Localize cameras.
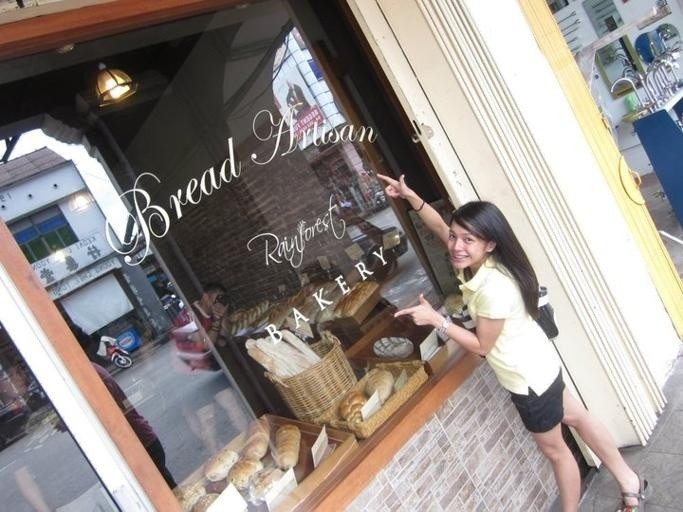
[214,294,231,306]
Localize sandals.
[615,471,653,512]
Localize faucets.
[609,32,678,109]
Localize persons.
[283,77,310,122]
[48,320,185,502]
[373,170,654,511]
[169,280,248,453]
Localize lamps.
[95,59,139,111]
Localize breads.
[366,370,395,407]
[372,335,415,359]
[173,418,301,512]
[253,280,383,336]
[338,391,367,424]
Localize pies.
[227,299,270,337]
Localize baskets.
[263,329,360,425]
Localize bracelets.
[414,200,426,213]
[210,324,219,332]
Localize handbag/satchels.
[459,286,558,359]
